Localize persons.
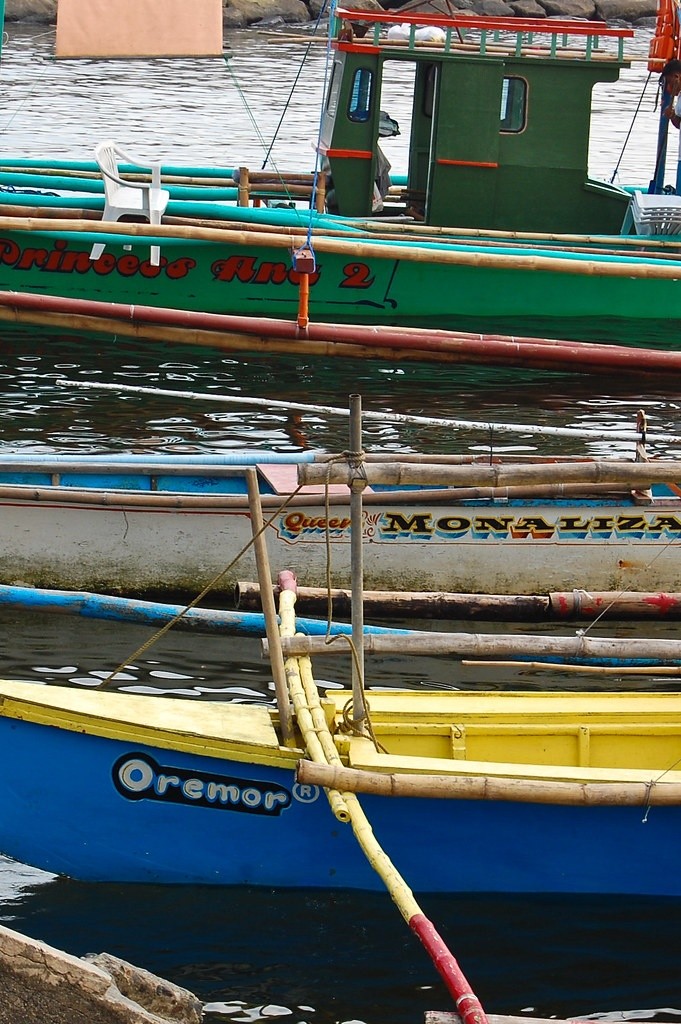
[658,60,681,196]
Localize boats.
[0,451,681,622]
[0,1,681,365]
[0,676,681,901]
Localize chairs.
[85,142,170,267]
[620,189,680,235]
